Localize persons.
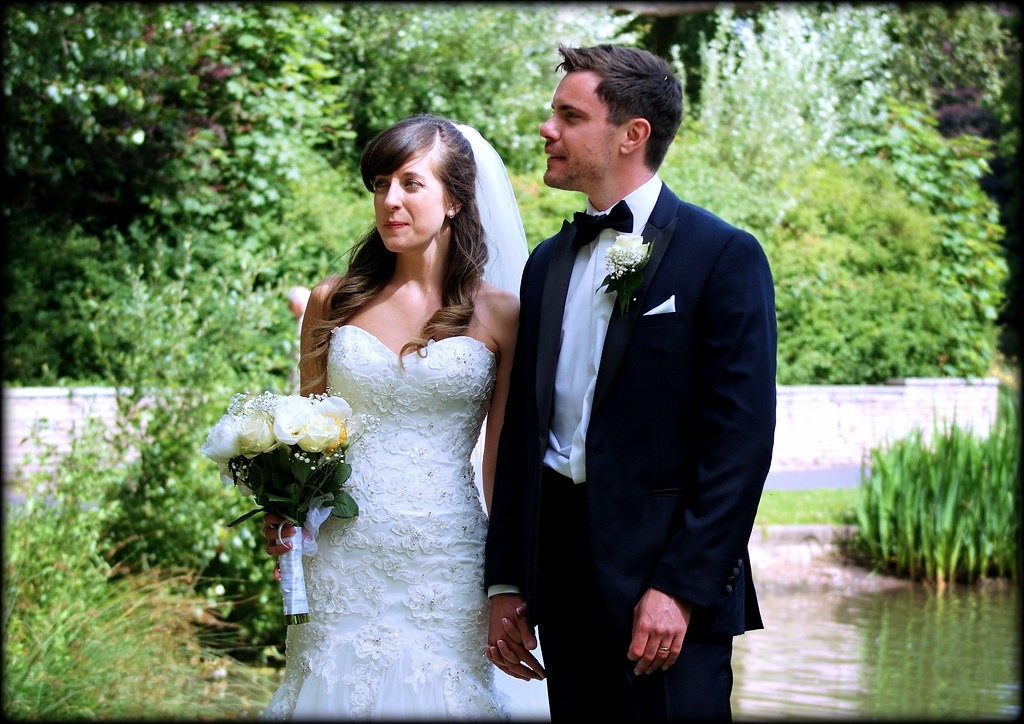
[485,45,777,723]
[266,113,534,724]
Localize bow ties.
[573,200,633,246]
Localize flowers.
[202,389,366,626]
[594,234,653,317]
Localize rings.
[659,647,669,653]
